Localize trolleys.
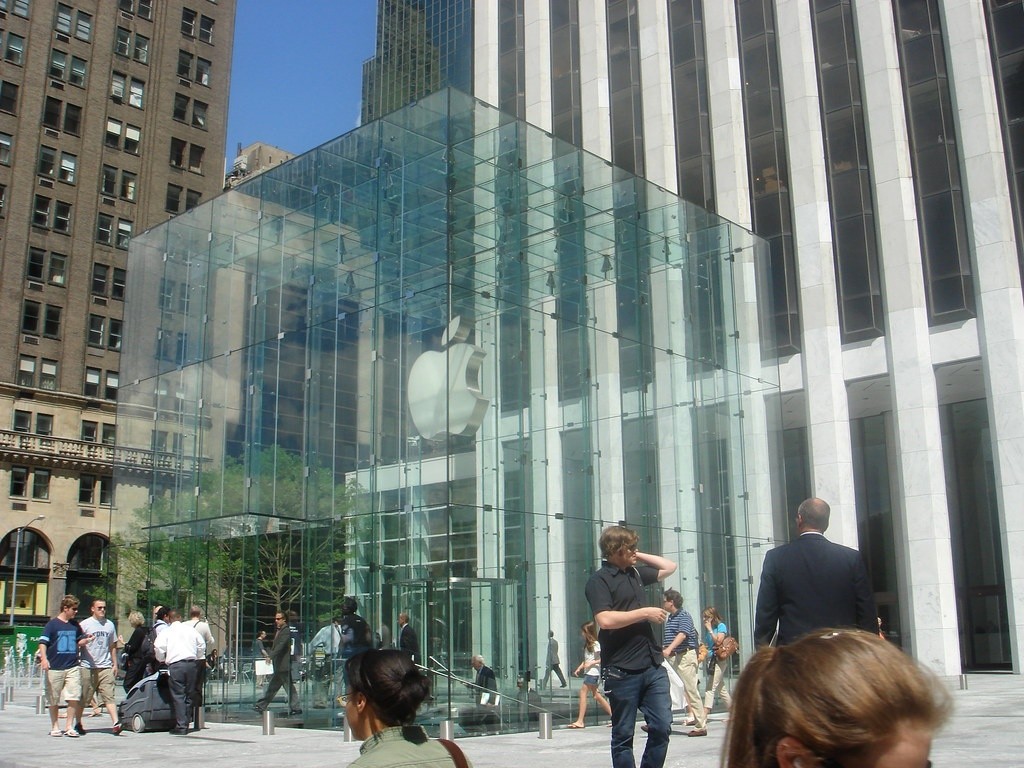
[115,663,176,733]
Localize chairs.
[236,663,253,685]
[221,663,238,684]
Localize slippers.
[566,723,585,728]
[606,723,612,727]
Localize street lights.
[8,514,47,626]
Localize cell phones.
[707,617,714,622]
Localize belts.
[669,647,693,656]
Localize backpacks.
[140,623,166,656]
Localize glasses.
[336,690,371,707]
[95,606,107,611]
[68,607,80,612]
[275,618,282,621]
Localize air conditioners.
[110,88,122,98]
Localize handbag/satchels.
[121,643,132,670]
[712,622,738,661]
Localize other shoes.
[481,730,500,736]
[684,716,694,723]
[64,728,79,737]
[169,728,188,735]
[688,729,707,737]
[468,732,481,736]
[249,705,265,715]
[687,720,707,725]
[286,710,302,716]
[560,683,567,687]
[313,705,326,708]
[88,707,102,717]
[74,723,86,734]
[113,721,128,736]
[641,725,648,732]
[49,729,62,736]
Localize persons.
[118,605,215,735]
[250,611,303,715]
[250,630,268,685]
[753,498,879,653]
[462,655,497,731]
[719,628,955,768]
[542,630,567,688]
[339,596,370,685]
[567,621,612,729]
[58,693,101,717]
[206,650,226,671]
[74,598,123,736]
[400,612,421,664]
[640,590,707,737]
[511,676,542,732]
[702,607,732,723]
[39,595,95,736]
[584,526,672,768]
[310,617,343,706]
[340,649,473,768]
